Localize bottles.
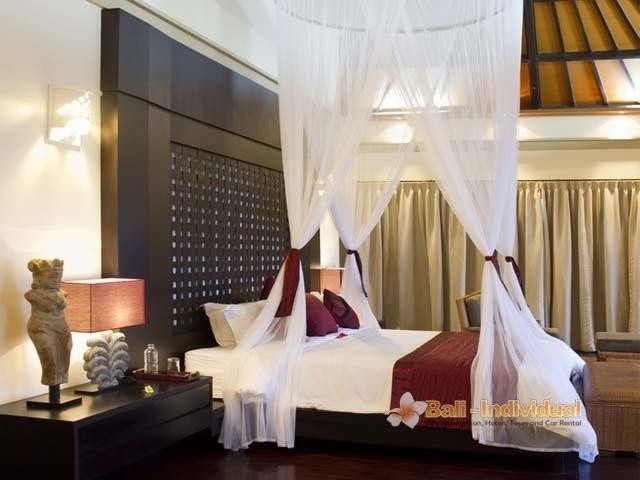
[144,343,158,372]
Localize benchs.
[586,357,640,454]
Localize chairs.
[455,291,561,340]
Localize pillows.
[223,296,270,344]
[321,287,361,330]
[201,299,238,348]
[303,292,340,337]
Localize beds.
[101,8,597,480]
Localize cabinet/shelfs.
[1,368,226,478]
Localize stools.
[595,331,640,361]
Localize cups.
[167,357,181,376]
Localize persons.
[23,258,73,387]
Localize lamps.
[59,275,147,398]
[45,79,97,151]
[318,267,344,292]
